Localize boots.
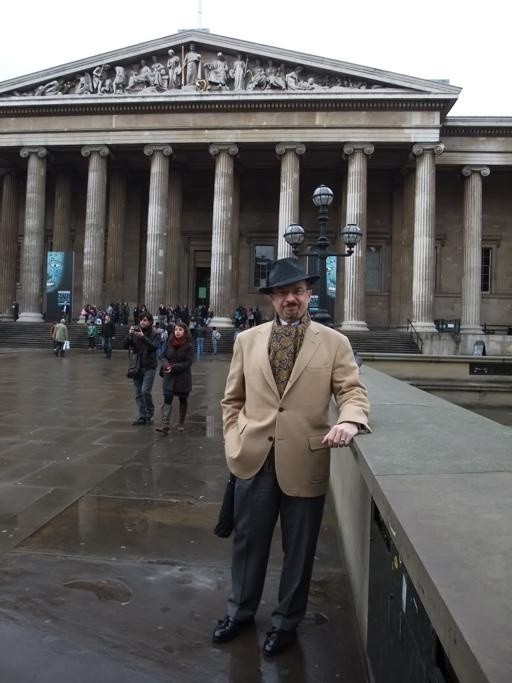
[155,405,172,434]
[176,404,186,432]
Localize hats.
[258,257,321,294]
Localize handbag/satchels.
[126,352,142,379]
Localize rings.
[340,438,345,441]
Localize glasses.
[276,288,306,296]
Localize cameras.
[134,326,141,332]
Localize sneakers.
[132,415,153,426]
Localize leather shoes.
[212,616,245,644]
[262,627,298,657]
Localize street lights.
[283,183,365,328]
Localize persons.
[51,302,261,360]
[39,43,329,97]
[123,311,161,427]
[12,301,19,322]
[212,258,373,657]
[155,323,194,435]
